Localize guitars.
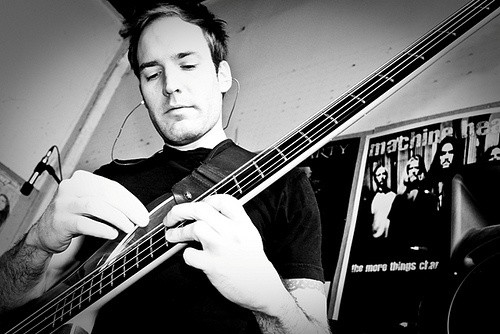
[1,0,499,331]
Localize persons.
[363,136,500,262]
[1,0,332,334]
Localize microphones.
[21,146,54,196]
